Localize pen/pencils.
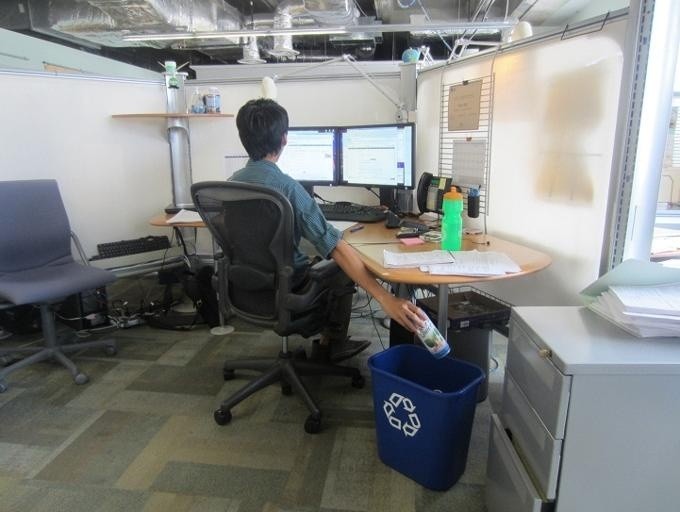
[467,184,482,196]
[350,225,364,233]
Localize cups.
[468,196,480,218]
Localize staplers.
[396,220,429,238]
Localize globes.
[402,46,419,63]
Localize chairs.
[1,177,124,393]
[190,180,367,435]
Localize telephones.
[416,172,453,213]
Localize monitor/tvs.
[274,125,339,198]
[338,121,416,217]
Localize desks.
[149,199,552,342]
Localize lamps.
[447,19,533,60]
[259,52,410,129]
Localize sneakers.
[312,337,371,365]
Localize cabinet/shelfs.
[485,306,680,512]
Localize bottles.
[441,185,464,250]
[191,87,205,113]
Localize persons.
[227,97,429,363]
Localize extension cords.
[117,311,160,329]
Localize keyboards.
[318,201,385,222]
[97,235,171,259]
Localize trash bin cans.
[367,344,486,492]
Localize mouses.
[385,216,400,228]
